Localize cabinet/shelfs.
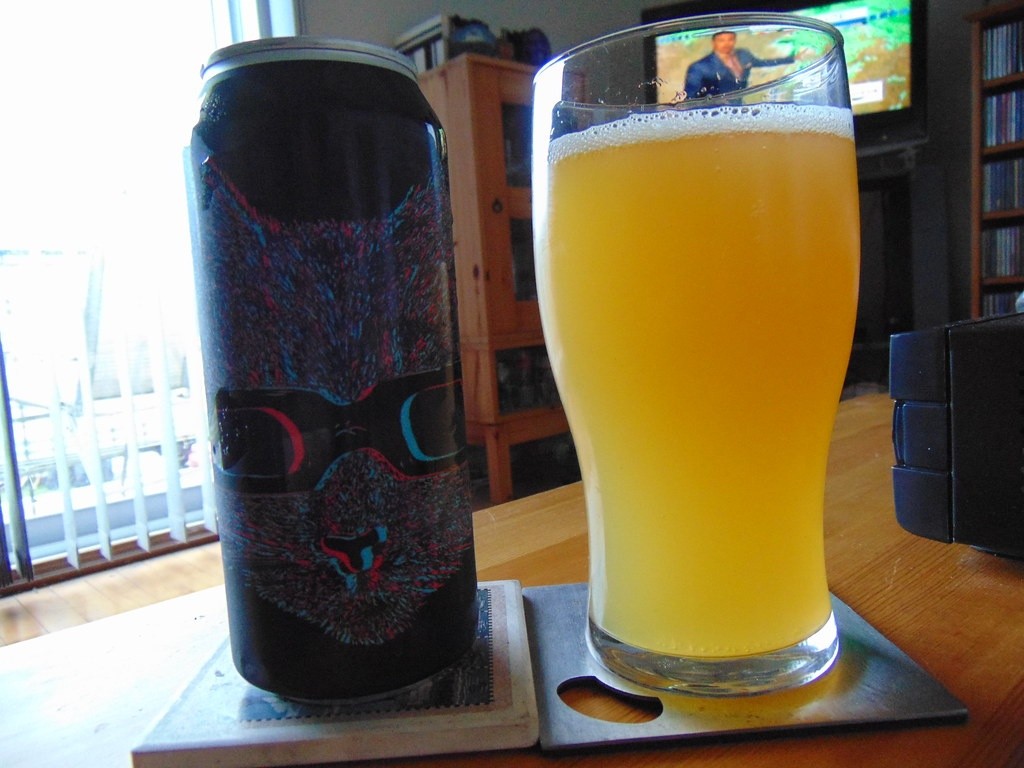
[961,0,1024,318]
[418,52,568,507]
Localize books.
[978,21,1024,317]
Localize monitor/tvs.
[641,0,927,156]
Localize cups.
[527,7,865,699]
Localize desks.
[0,394,1024,768]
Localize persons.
[684,30,809,112]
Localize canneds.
[189,35,478,705]
